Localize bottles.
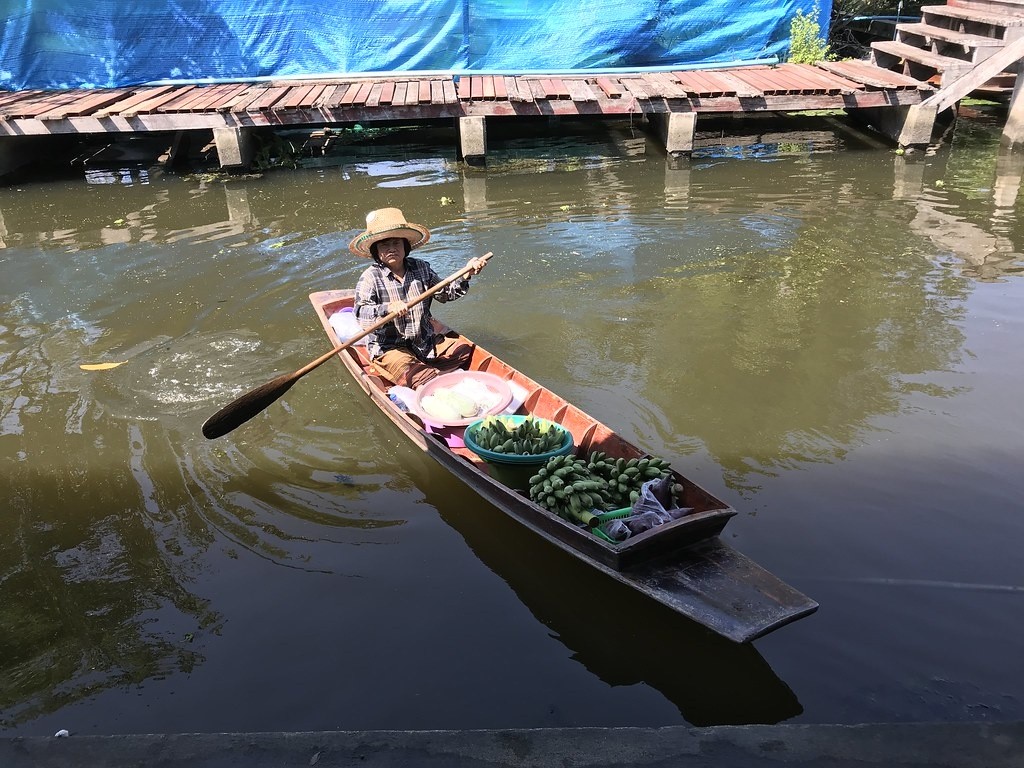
[390,394,410,413]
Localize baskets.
[592,507,632,544]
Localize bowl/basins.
[463,413,574,490]
[416,370,513,429]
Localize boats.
[310,289,821,648]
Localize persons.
[349,208,487,391]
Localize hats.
[349,208,430,259]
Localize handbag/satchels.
[605,478,680,542]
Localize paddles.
[201,250,495,440]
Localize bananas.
[475,411,566,455]
[528,451,676,515]
